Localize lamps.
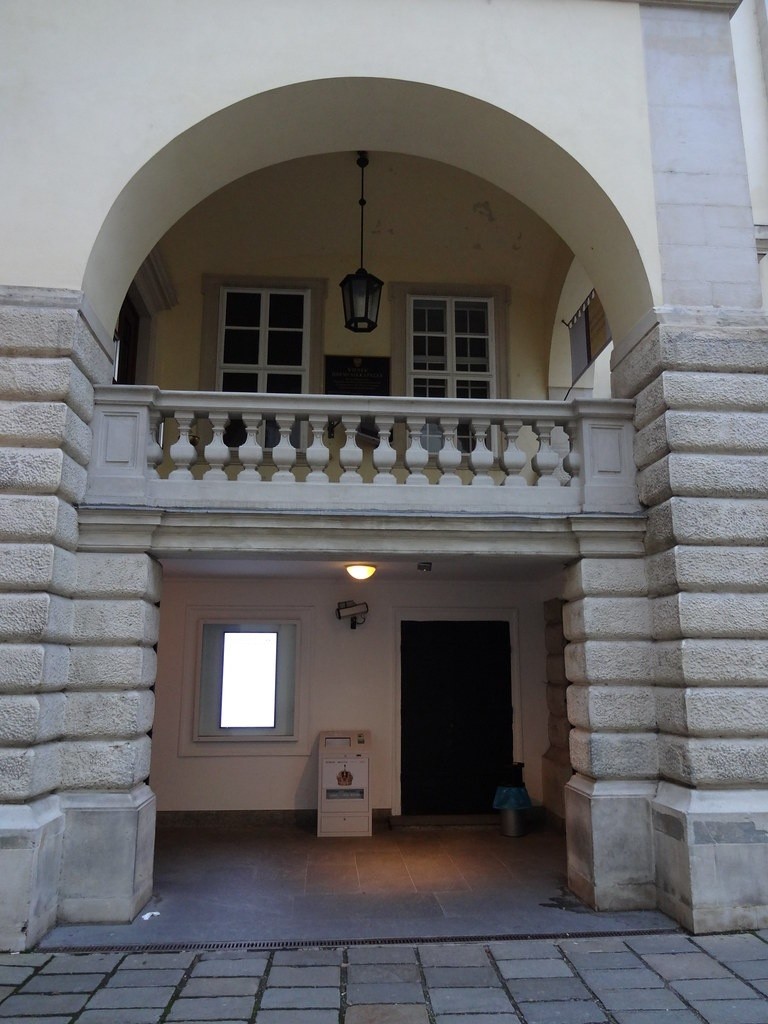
[344,564,377,580]
[340,151,385,333]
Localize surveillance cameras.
[334,601,369,620]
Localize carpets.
[388,815,504,828]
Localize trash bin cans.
[493,785,534,838]
